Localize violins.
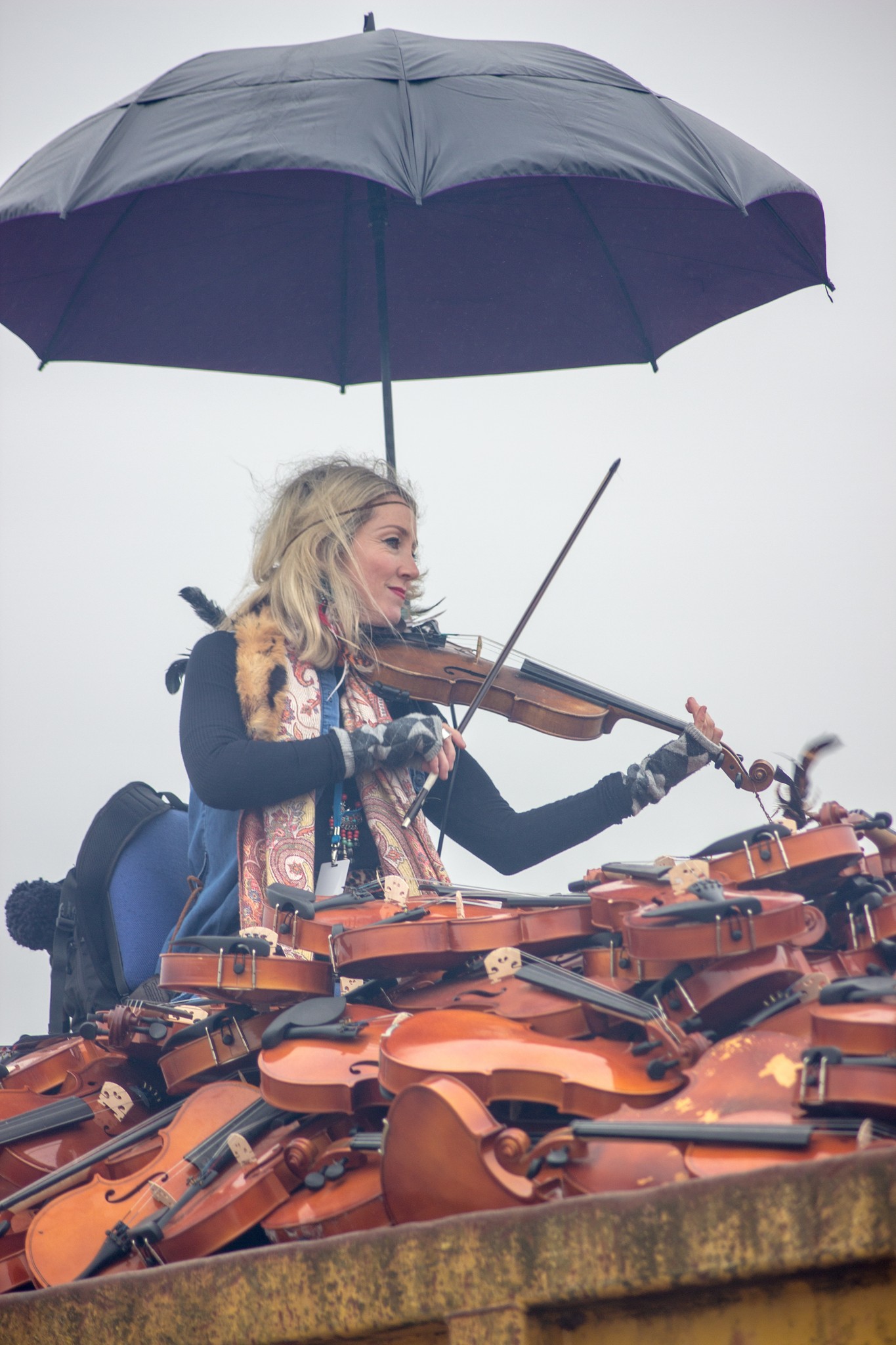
[1,730,894,1294]
[363,632,775,795]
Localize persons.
[156,454,726,971]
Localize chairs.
[47,780,189,1044]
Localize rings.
[442,728,452,740]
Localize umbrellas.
[1,13,835,484]
